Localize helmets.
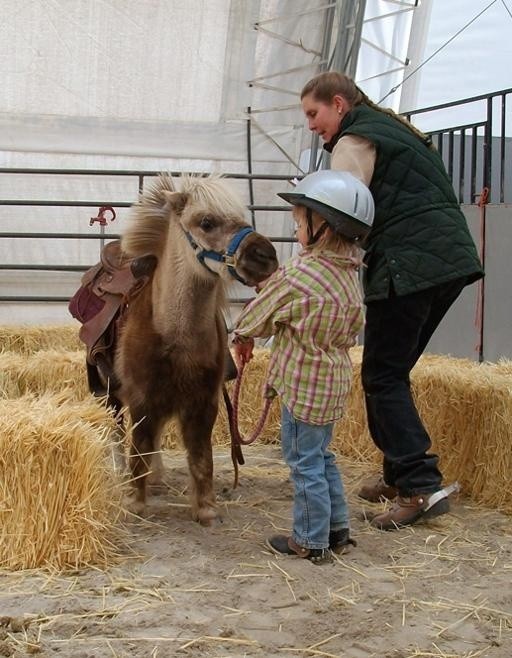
[274,170,376,244]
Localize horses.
[86,168,278,528]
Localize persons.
[229,168,376,563]
[254,70,485,528]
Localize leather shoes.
[356,470,450,531]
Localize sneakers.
[265,522,350,565]
